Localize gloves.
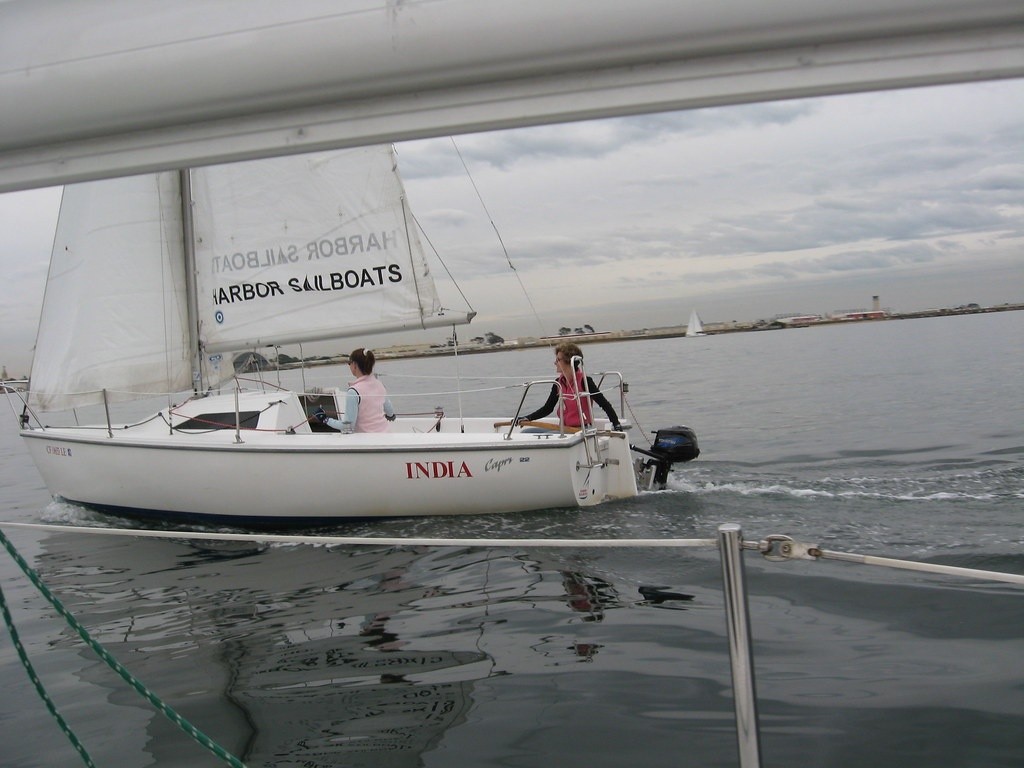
[511,416,529,428]
[615,424,623,432]
[312,407,329,424]
[385,414,396,421]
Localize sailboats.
[0,143,700,517]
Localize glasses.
[556,357,563,362]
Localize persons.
[513,343,623,432]
[313,349,395,433]
